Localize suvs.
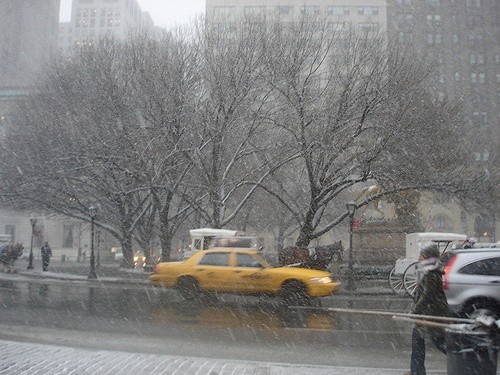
[431,247,500,343]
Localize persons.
[306,253,323,271]
[464,240,472,249]
[404,243,453,375]
[41,240,52,271]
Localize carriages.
[188,227,345,276]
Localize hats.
[420,245,440,259]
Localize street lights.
[88,204,99,278]
[26,215,38,270]
[346,198,357,291]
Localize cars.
[146,247,343,309]
